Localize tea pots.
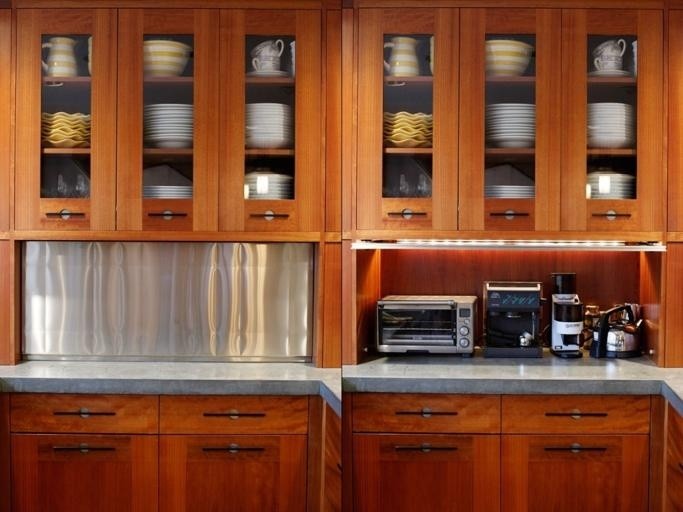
[41,38,80,76]
[589,305,645,358]
[383,37,420,78]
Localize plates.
[588,70,630,76]
[486,181,534,195]
[586,171,632,197]
[246,70,289,78]
[244,171,296,199]
[244,104,294,149]
[146,181,190,200]
[145,103,194,151]
[587,102,632,147]
[384,110,435,147]
[485,104,535,148]
[44,106,93,147]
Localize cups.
[250,39,285,70]
[592,39,627,70]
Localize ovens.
[377,294,477,357]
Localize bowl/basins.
[485,39,534,78]
[142,40,192,77]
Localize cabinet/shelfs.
[341,347,683,512]
[2,2,342,241]
[0,361,341,512]
[342,1,682,244]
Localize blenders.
[551,270,584,359]
